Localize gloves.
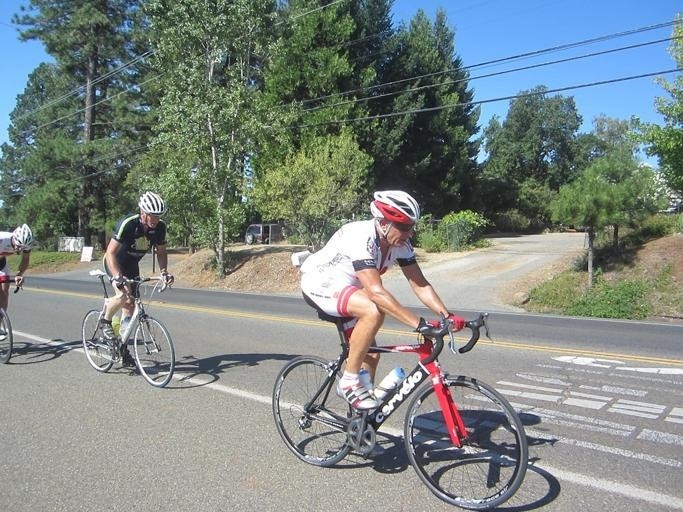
[427,319,441,328]
[448,313,465,329]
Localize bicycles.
[82,269,175,388]
[0,280,24,363]
[272,309,528,511]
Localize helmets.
[12,223,33,251]
[138,191,166,216]
[370,190,421,225]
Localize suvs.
[244,223,284,244]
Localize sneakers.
[123,355,136,366]
[99,319,115,339]
[361,440,385,455]
[337,380,379,409]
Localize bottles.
[119,315,132,338]
[358,367,373,397]
[373,366,408,400]
[111,313,120,336]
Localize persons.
[300,189,466,457]
[99,191,175,371]
[0,223,35,342]
